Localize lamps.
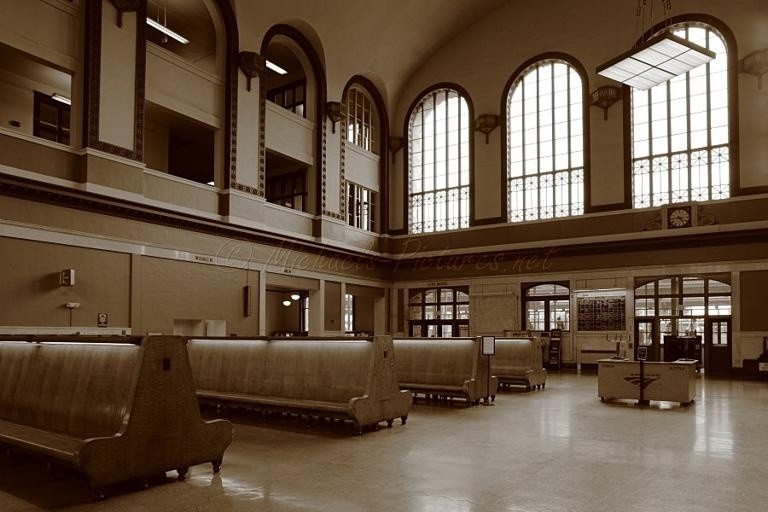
[326,101,348,135]
[239,51,263,92]
[474,113,499,144]
[388,136,405,164]
[593,2,716,123]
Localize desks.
[575,342,625,376]
[594,357,699,407]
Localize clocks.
[665,204,692,230]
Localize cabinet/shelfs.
[664,334,703,372]
